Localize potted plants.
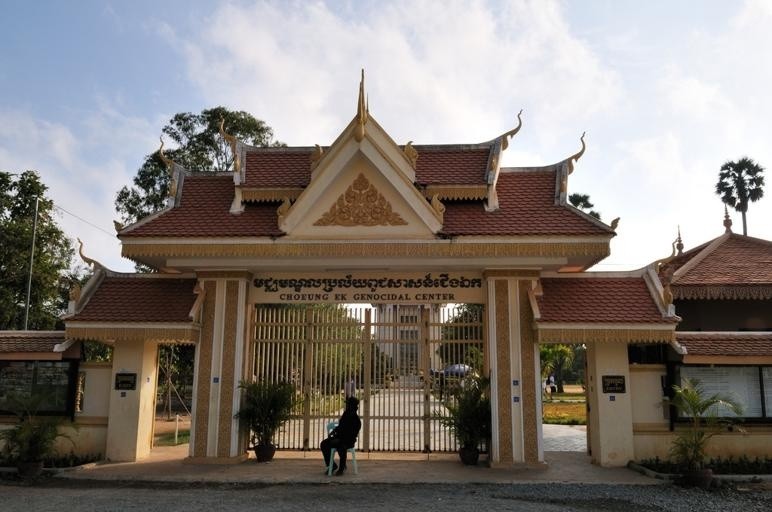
[428,375,490,465]
[0,388,80,477]
[232,378,306,461]
[654,378,748,489]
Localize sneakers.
[337,465,346,476]
[326,464,338,475]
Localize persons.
[550,372,556,400]
[542,376,548,400]
[345,376,354,398]
[436,373,449,403]
[320,397,361,477]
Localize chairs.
[323,422,357,476]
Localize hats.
[343,397,360,410]
